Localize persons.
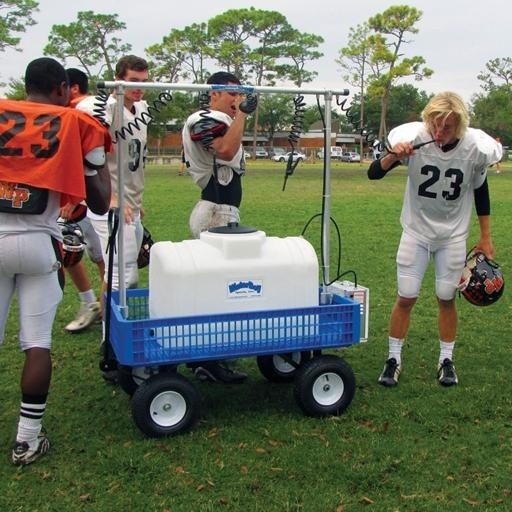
[367,89,504,387]
[59,67,104,332]
[87,56,152,342]
[495,137,500,174]
[0,55,112,467]
[181,71,249,385]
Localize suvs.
[316,146,344,160]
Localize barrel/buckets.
[148,221,320,350]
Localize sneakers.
[376,357,403,387]
[193,361,249,386]
[10,432,53,466]
[435,356,459,386]
[64,296,103,332]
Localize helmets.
[55,219,89,268]
[457,251,505,308]
[135,227,157,270]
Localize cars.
[339,151,360,164]
[242,145,307,162]
[503,146,512,160]
